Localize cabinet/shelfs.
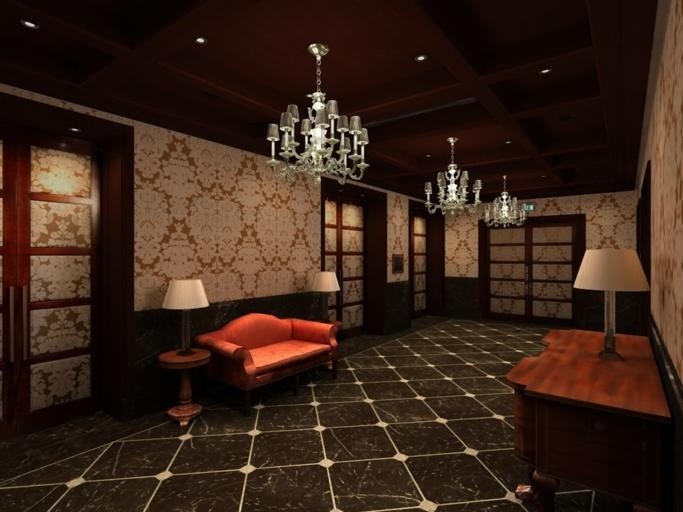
[505,328,671,501]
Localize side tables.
[158,349,210,421]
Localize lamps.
[161,278,210,356]
[267,41,371,186]
[308,271,341,324]
[423,137,482,218]
[573,249,651,363]
[479,174,526,229]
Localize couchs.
[193,312,339,419]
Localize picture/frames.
[392,254,404,273]
